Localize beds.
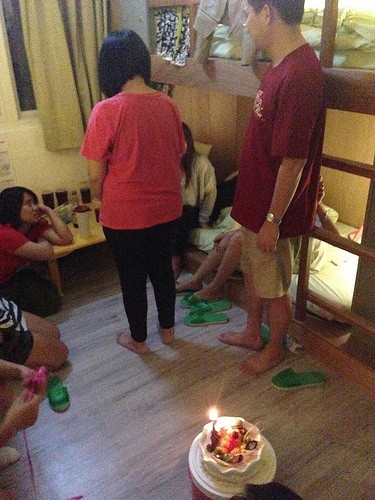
[147,1,375,399]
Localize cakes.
[196,417,266,483]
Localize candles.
[207,408,217,431]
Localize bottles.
[75,206,92,238]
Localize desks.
[47,202,107,297]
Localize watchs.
[266,213,281,224]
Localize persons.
[216,0,328,375]
[0,297,69,372]
[175,175,340,302]
[0,360,47,469]
[170,121,217,280]
[0,186,73,318]
[79,29,188,355]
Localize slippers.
[271,367,327,390]
[175,280,232,326]
[46,375,71,412]
[261,325,270,343]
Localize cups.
[80,187,92,204]
[55,188,68,206]
[70,205,79,229]
[41,190,55,210]
